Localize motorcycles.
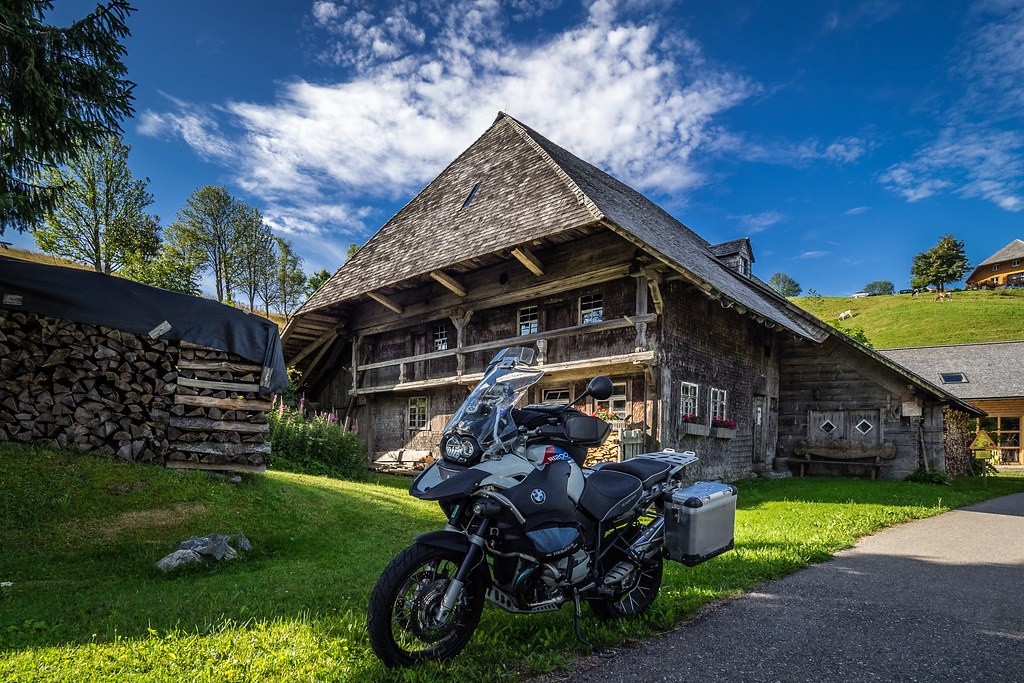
[366,346,737,672]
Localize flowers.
[593,406,619,420]
[682,413,707,426]
[713,416,736,429]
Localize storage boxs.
[661,482,738,568]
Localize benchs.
[788,440,896,481]
